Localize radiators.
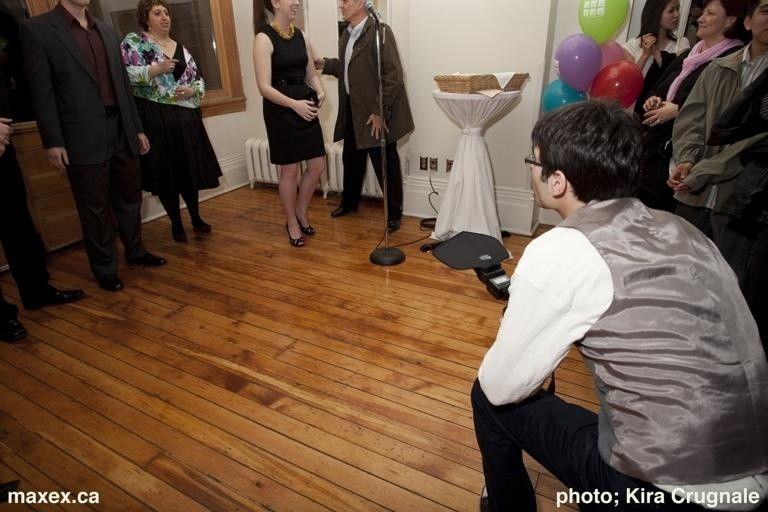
[245,137,407,199]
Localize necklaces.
[150,32,167,47]
[270,21,295,41]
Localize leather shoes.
[388,220,400,233]
[286,222,304,247]
[127,253,167,266]
[331,205,359,217]
[295,217,314,236]
[96,274,124,291]
[21,287,84,310]
[173,219,211,241]
[0,298,29,341]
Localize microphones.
[364,0,384,27]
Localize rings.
[181,91,184,95]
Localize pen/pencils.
[163,53,170,59]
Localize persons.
[621,0,690,117]
[638,1,746,204]
[118,0,223,243]
[314,1,414,233]
[25,0,168,291]
[666,1,768,348]
[0,41,84,343]
[470,101,767,509]
[252,0,326,248]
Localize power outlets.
[419,156,428,170]
[430,158,438,171]
[446,159,453,171]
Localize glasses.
[525,153,541,166]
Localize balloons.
[576,0,628,44]
[554,34,599,95]
[540,82,591,114]
[586,38,623,94]
[591,61,646,109]
